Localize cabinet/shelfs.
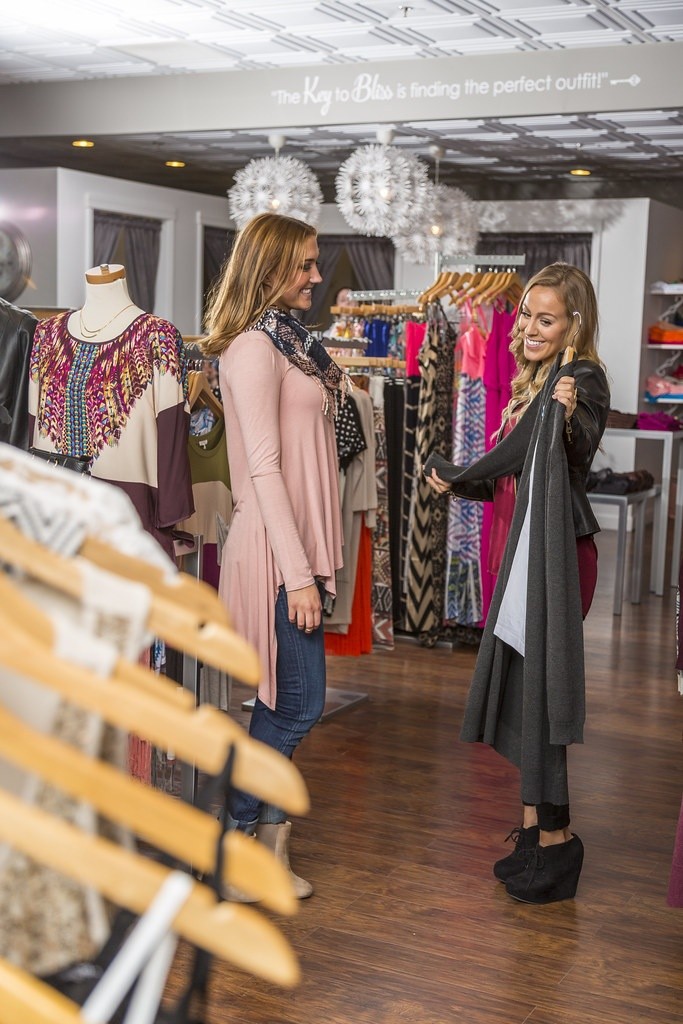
[642,289,683,405]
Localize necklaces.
[80,304,136,338]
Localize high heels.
[493,820,542,884]
[506,835,584,906]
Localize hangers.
[329,255,526,379]
[182,340,224,418]
[558,312,581,367]
[1,456,311,1024]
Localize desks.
[602,429,683,595]
[586,485,661,616]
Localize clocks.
[0,220,32,302]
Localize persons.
[193,214,342,902]
[423,263,611,902]
[336,288,360,318]
[28,264,195,561]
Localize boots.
[207,811,258,903]
[253,821,312,899]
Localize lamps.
[335,128,433,237]
[389,146,479,263]
[227,133,326,233]
